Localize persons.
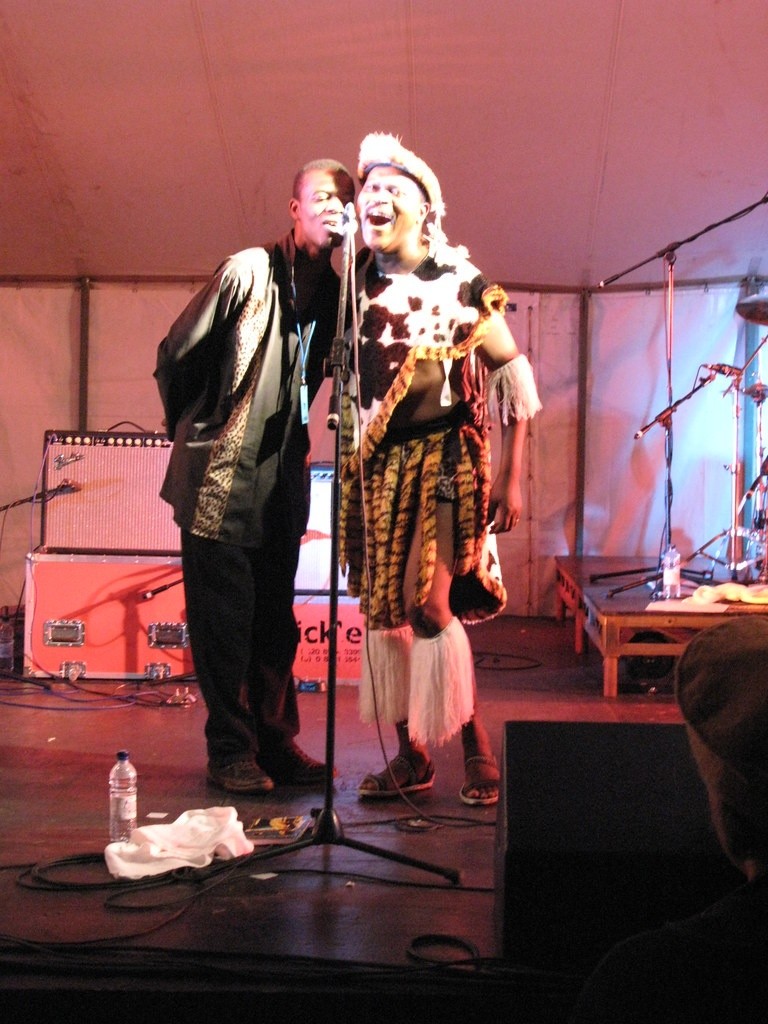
[153,158,355,790]
[335,132,543,806]
[572,618,768,1024]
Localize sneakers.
[256,742,336,788]
[207,757,273,793]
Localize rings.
[516,518,519,522]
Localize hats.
[358,128,445,226]
[675,614,768,794]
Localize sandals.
[359,755,435,796]
[459,752,501,804]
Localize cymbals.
[736,294,768,326]
[721,384,768,395]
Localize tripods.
[587,191,768,599]
[191,223,463,885]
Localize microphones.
[63,478,83,492]
[703,363,741,377]
[336,202,359,236]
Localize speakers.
[40,430,183,557]
[292,464,351,596]
[493,720,749,961]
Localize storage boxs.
[23,429,196,681]
[291,594,366,687]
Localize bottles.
[0,616,14,672]
[663,544,681,598]
[108,750,138,844]
